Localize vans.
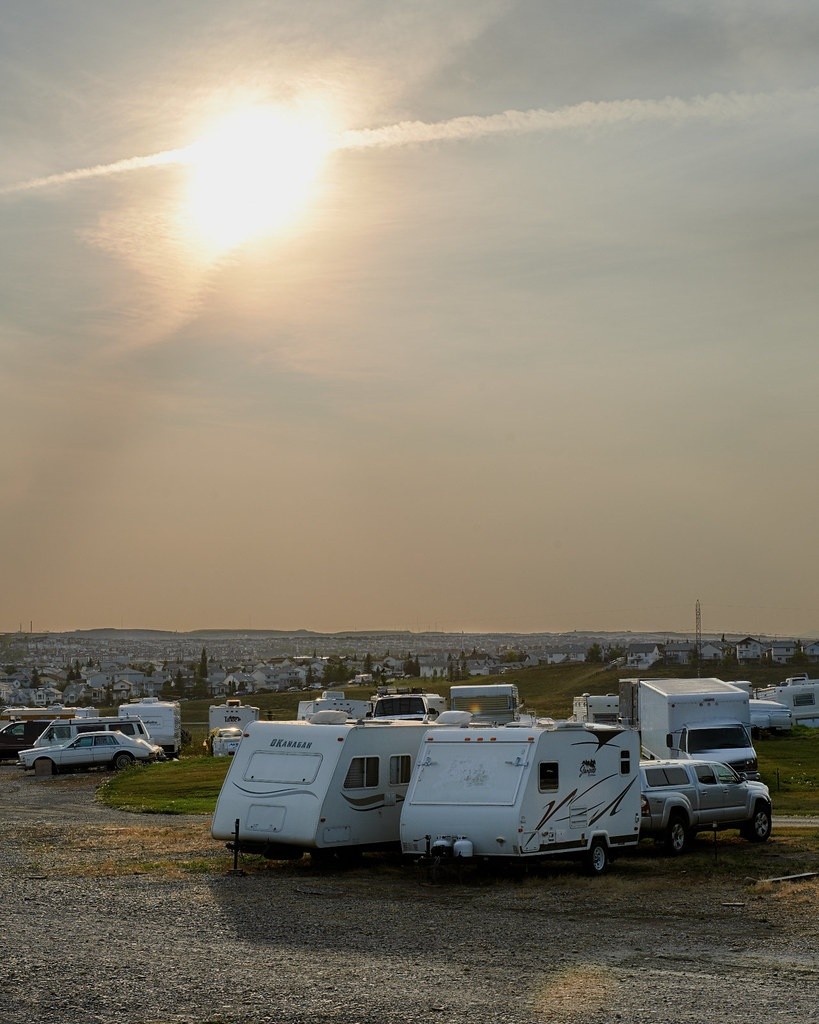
[32,715,150,748]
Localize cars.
[232,691,246,696]
[303,686,314,691]
[348,680,355,684]
[0,720,53,762]
[318,686,329,690]
[16,731,157,775]
[254,688,269,694]
[212,729,243,752]
[214,693,225,698]
[174,698,189,702]
[0,702,64,709]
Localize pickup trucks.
[287,686,303,692]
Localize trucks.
[355,673,374,684]
[366,694,436,721]
[638,678,761,782]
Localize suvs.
[639,759,773,858]
